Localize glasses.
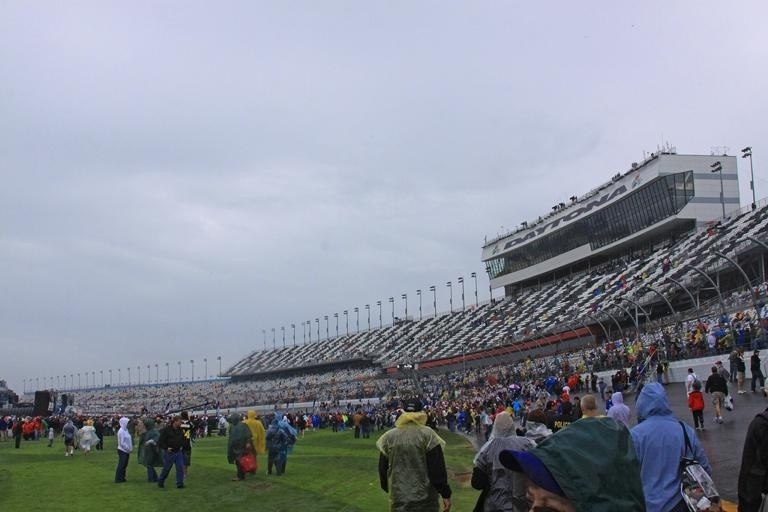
[511,495,560,512]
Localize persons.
[224,305,471,440]
[241,410,265,476]
[265,418,288,476]
[1,413,229,457]
[470,200,766,511]
[115,416,132,483]
[226,409,259,481]
[274,409,299,473]
[157,415,193,487]
[136,417,162,481]
[18,381,224,420]
[375,397,453,512]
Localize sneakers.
[718,418,724,424]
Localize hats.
[498,448,567,495]
[403,398,425,412]
[715,361,721,364]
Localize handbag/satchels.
[674,418,724,512]
[237,448,257,473]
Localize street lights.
[711,160,725,219]
[741,145,756,206]
[260,292,410,353]
[457,276,466,310]
[416,289,422,320]
[23,355,223,394]
[429,285,437,316]
[446,281,454,312]
[471,271,478,307]
[484,266,493,301]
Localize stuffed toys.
[178,410,191,478]
